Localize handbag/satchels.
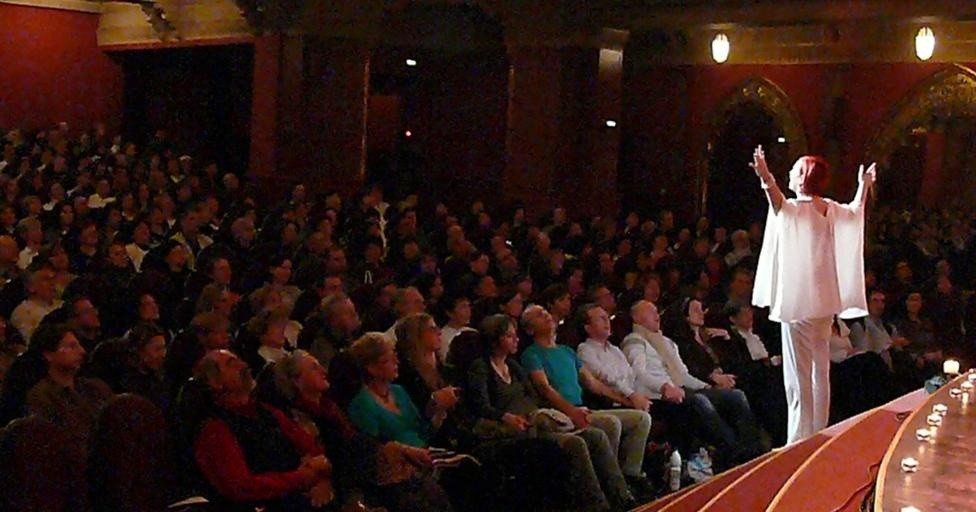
[530,408,576,434]
[646,440,675,476]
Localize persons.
[0,112,975,512]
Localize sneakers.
[618,468,661,509]
[721,439,771,462]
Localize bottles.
[699,446,709,463]
[663,442,683,490]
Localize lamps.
[711,29,735,66]
[912,20,937,64]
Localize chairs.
[0,415,82,512]
[85,392,213,512]
